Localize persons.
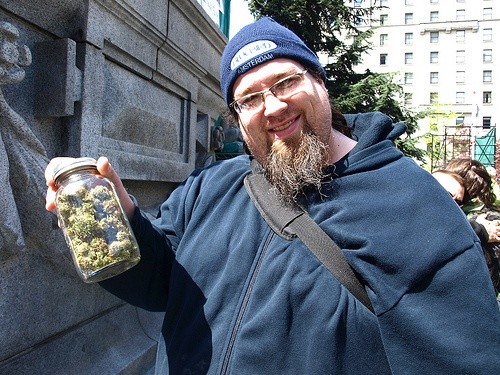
[45,15,498,374]
[429,155,499,244]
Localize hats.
[220,15,327,115]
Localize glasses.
[229,66,311,114]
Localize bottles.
[50,156,141,284]
